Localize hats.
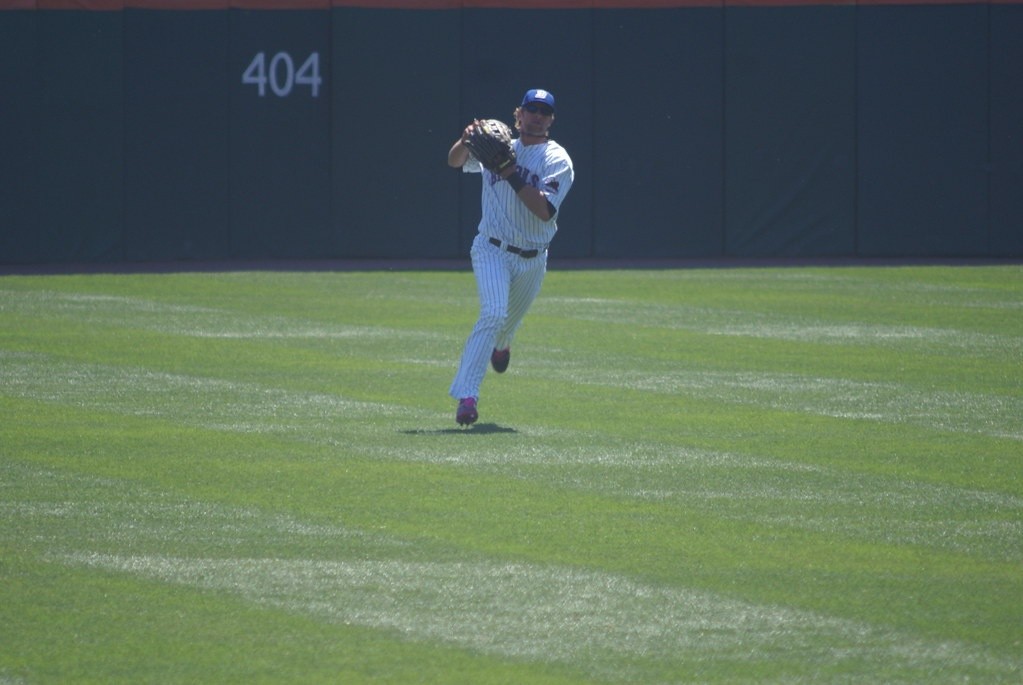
[520,89,556,113]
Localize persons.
[449,89,577,427]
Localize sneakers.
[491,349,509,372]
[456,398,478,426]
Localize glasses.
[525,105,551,116]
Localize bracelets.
[506,172,527,194]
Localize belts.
[489,237,538,258]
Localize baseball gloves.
[464,119,516,174]
